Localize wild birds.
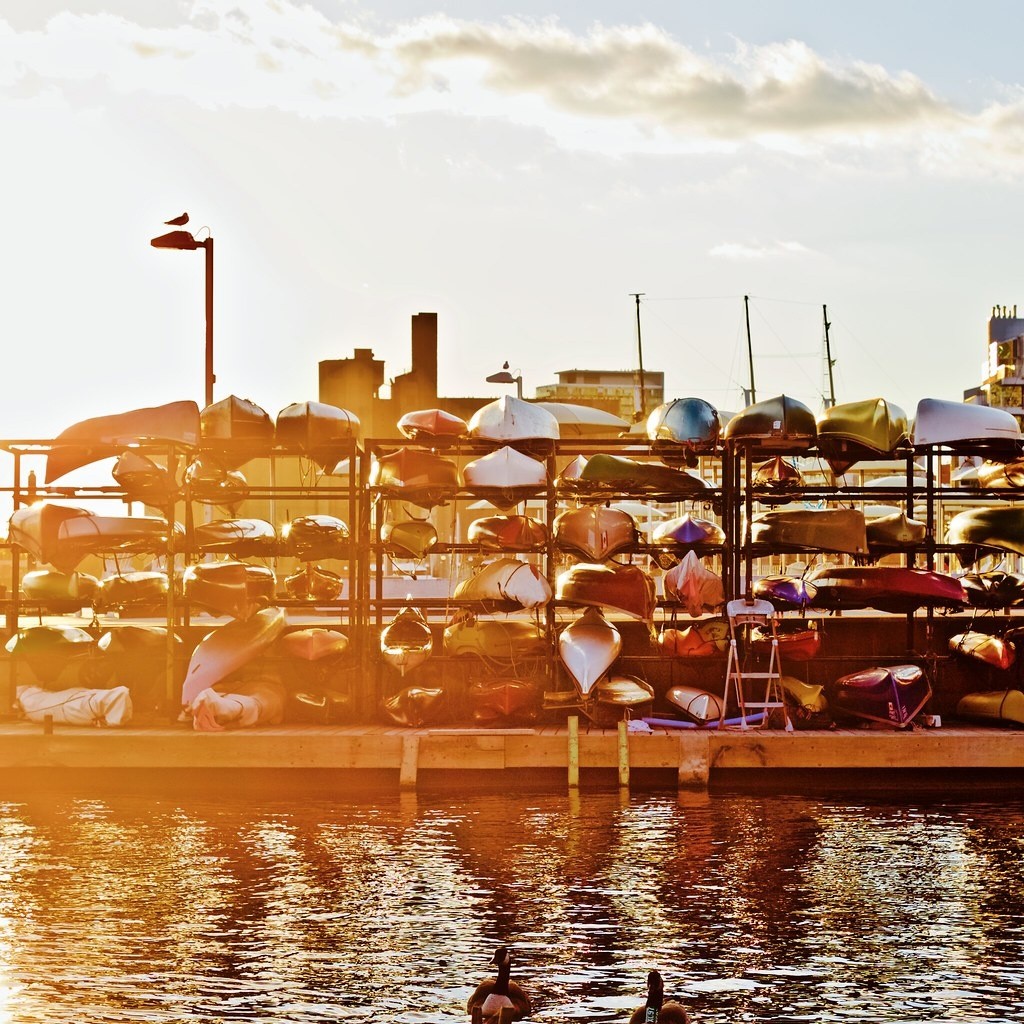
[164,212,189,230]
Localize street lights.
[629,290,651,422]
[149,225,218,407]
[486,374,523,398]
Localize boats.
[8,395,1024,727]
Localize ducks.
[629,970,692,1024]
[467,948,532,1018]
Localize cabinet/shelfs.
[0,429,1024,725]
